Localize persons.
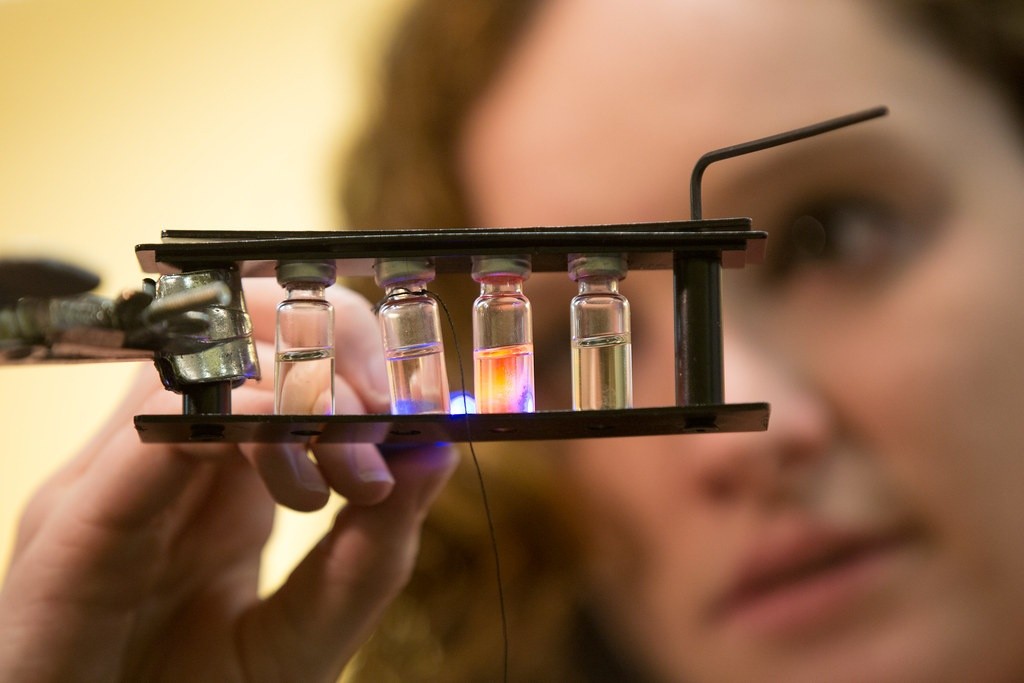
[0,0,1024,683]
